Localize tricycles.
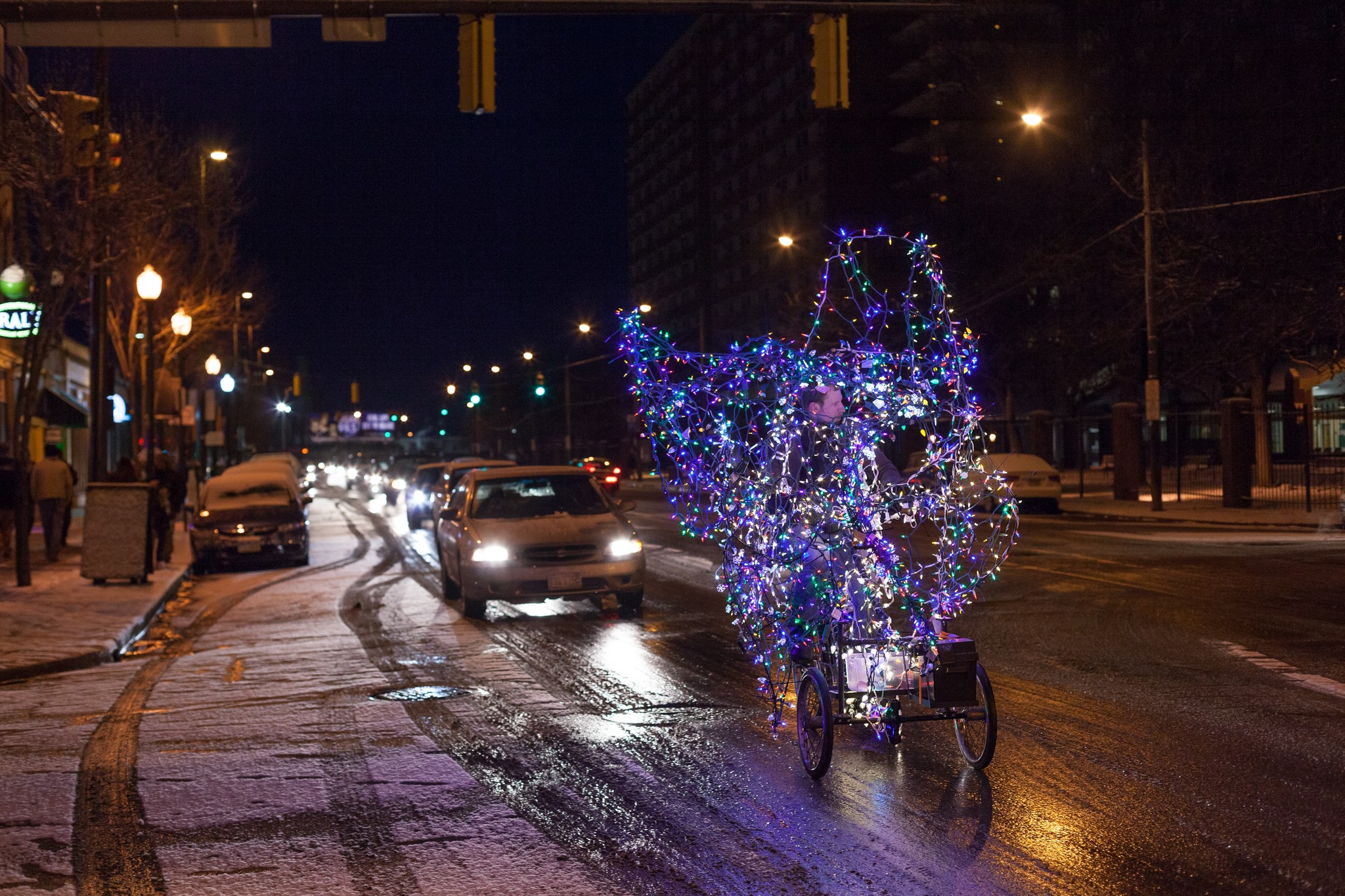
[773,495,998,782]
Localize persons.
[0,436,206,569]
[768,381,910,668]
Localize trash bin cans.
[80,483,156,583]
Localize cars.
[955,454,1061,512]
[314,456,645,621]
[184,452,324,574]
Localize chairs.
[476,488,521,517]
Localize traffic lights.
[470,382,482,406]
[93,123,121,200]
[534,373,546,401]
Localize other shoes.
[785,630,815,665]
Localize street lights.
[134,264,162,571]
[195,149,290,485]
[640,294,706,404]
[204,354,222,479]
[219,374,236,470]
[779,234,801,344]
[1023,112,1166,509]
[169,310,192,388]
